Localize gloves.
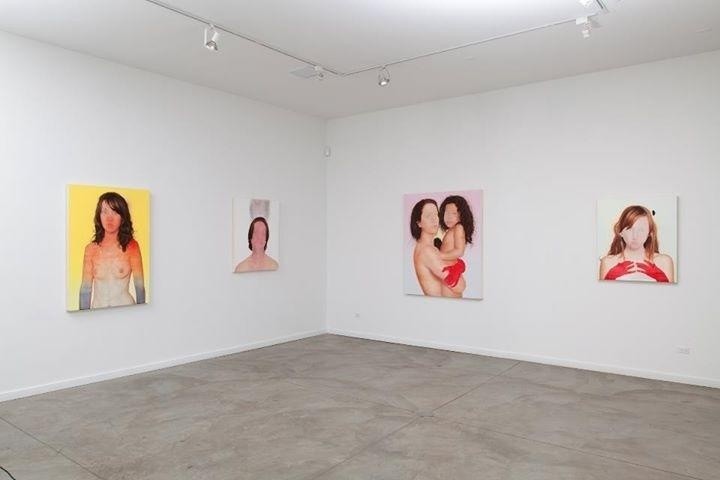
[442,259,465,288]
[636,260,669,282]
[604,261,635,279]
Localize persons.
[439,195,474,299]
[77,193,146,309]
[410,199,466,299]
[234,218,280,271]
[599,204,676,282]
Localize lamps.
[201,23,223,53]
[376,62,390,88]
[313,66,328,82]
